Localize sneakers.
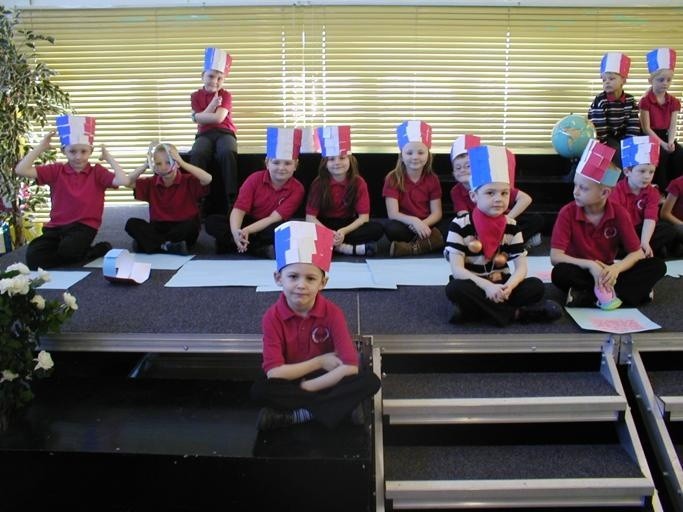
[641,288,656,304]
[565,288,584,306]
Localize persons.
[306,125,383,257]
[14,115,124,270]
[443,133,564,324]
[384,119,454,258]
[249,221,380,432]
[190,46,239,206]
[588,51,641,182]
[550,136,683,309]
[127,144,213,256]
[637,47,683,197]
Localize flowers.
[0,260,78,430]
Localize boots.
[390,227,444,257]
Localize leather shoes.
[167,241,189,254]
[448,306,463,323]
[349,402,364,425]
[257,407,286,433]
[520,300,561,323]
[94,242,111,257]
[133,240,144,253]
[262,245,274,258]
[353,240,377,257]
